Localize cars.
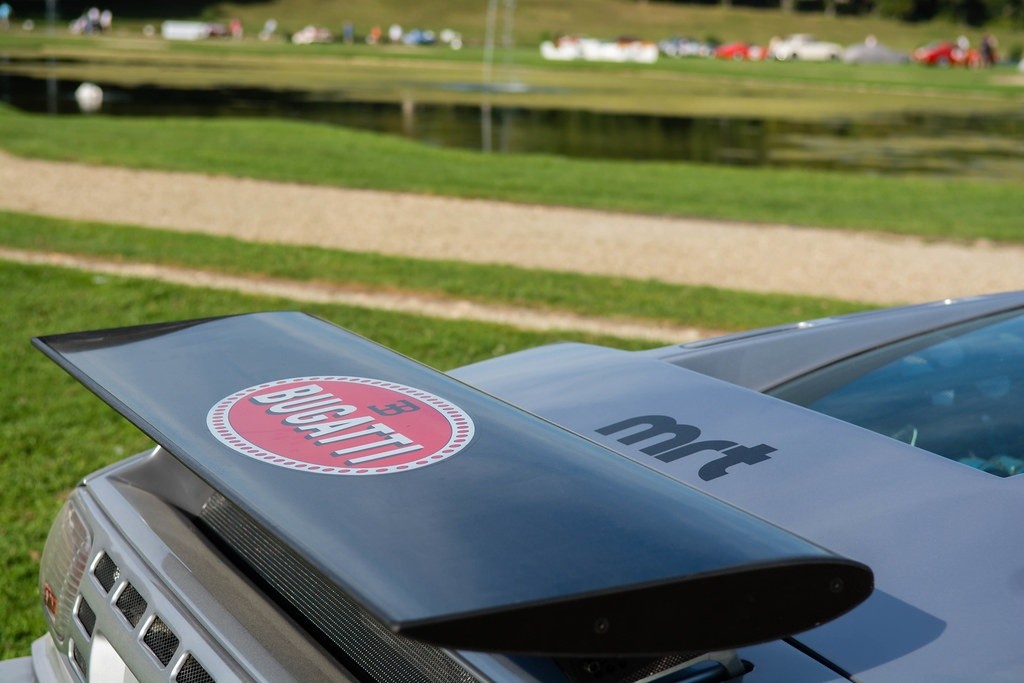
[0,274,1024,683]
[72,8,1005,78]
